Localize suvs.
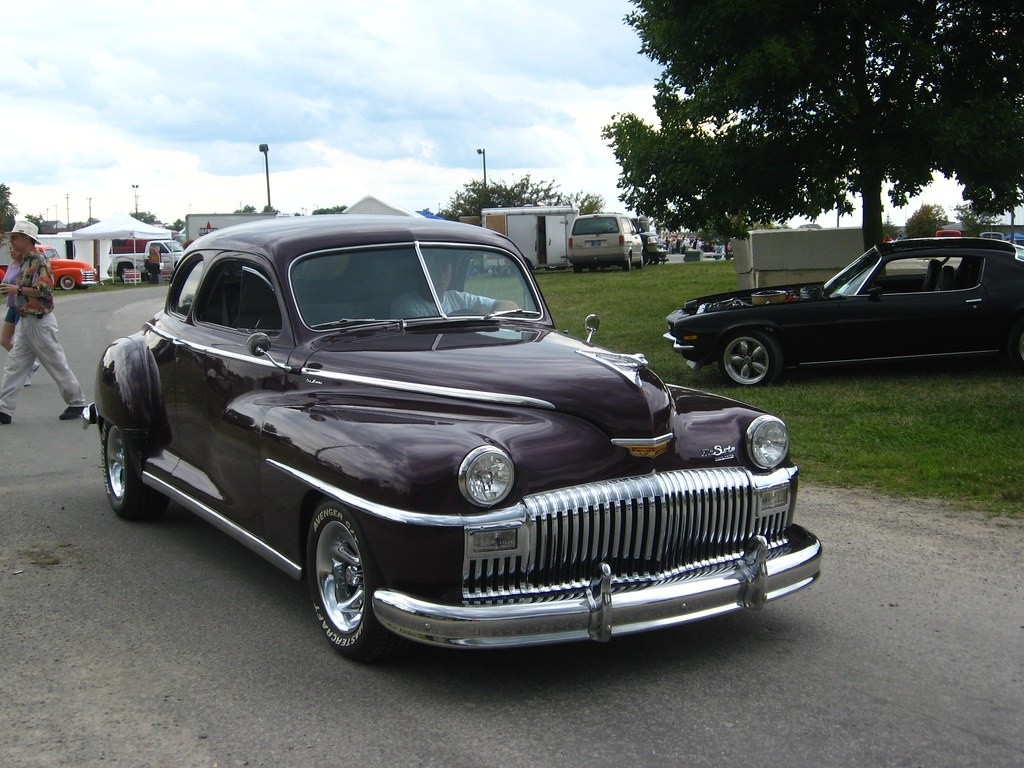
[567,213,645,274]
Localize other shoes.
[59,407,86,420]
[33,362,39,372]
[24,382,30,386]
[0,412,11,424]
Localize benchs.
[202,291,370,325]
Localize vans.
[82,214,824,664]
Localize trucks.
[113,240,186,283]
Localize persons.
[0,221,84,423]
[664,237,732,254]
[391,257,517,319]
[883,229,905,242]
[145,245,161,284]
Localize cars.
[661,237,1024,389]
[936,228,1005,241]
[0,246,100,291]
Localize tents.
[72,212,174,285]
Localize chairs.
[231,279,282,329]
[920,259,942,292]
[935,266,955,291]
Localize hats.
[5,220,42,245]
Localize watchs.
[17,286,22,294]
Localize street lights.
[132,184,139,220]
[259,143,270,208]
[477,148,487,189]
